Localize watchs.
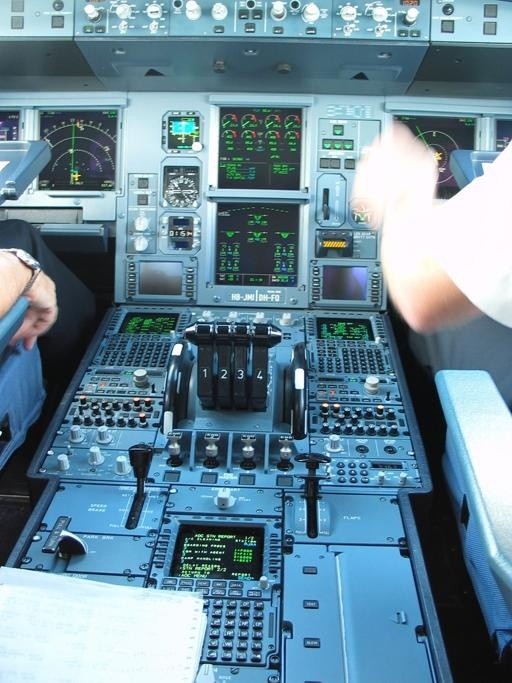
[1,245,44,296]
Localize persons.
[0,218,104,389]
[351,118,512,409]
